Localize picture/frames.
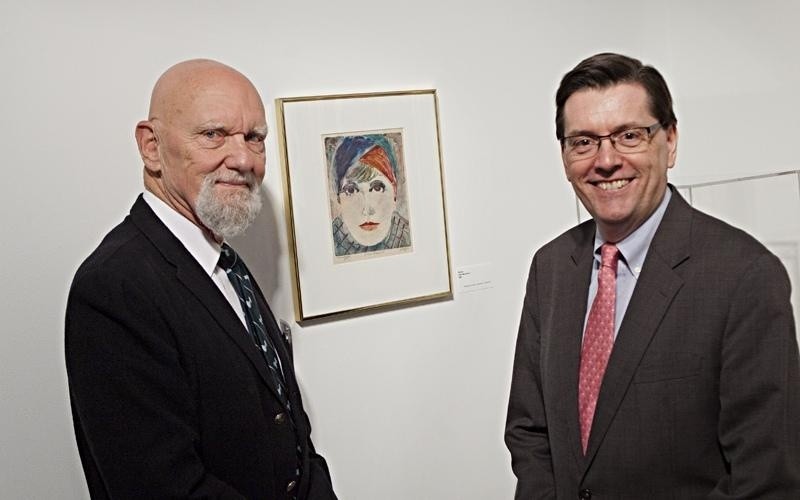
[274,88,453,325]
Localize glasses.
[560,122,661,162]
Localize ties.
[216,242,291,414]
[577,242,618,458]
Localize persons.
[321,133,410,255]
[502,52,800,500]
[64,55,340,500]
